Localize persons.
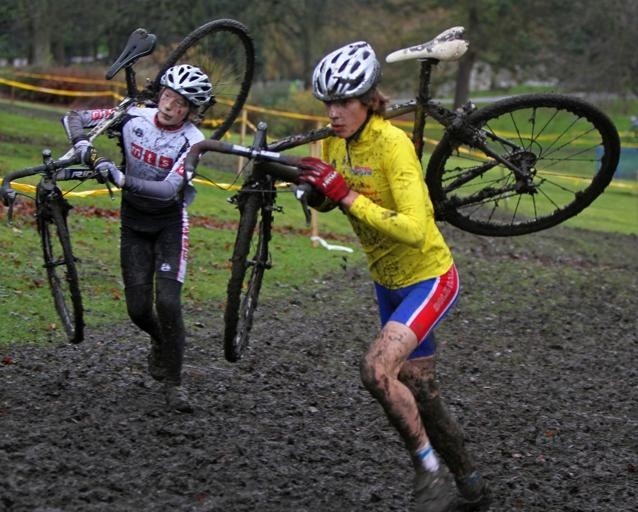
[61,63,216,416]
[291,40,486,511]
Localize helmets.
[311,40,379,103]
[157,65,214,107]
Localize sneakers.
[149,338,194,412]
[407,468,491,511]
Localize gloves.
[72,139,96,165]
[94,157,122,190]
[295,152,350,203]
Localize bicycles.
[2,18,256,345]
[185,27,621,362]
